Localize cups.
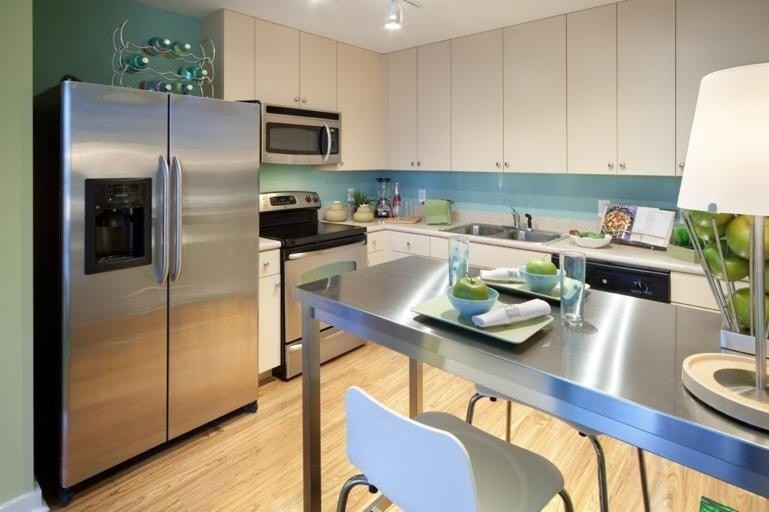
[560,251,586,329]
[446,235,470,287]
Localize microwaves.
[260,103,342,166]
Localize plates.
[409,284,555,345]
[474,276,592,303]
[568,232,613,249]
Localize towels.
[478,265,521,284]
[424,199,452,225]
[472,297,551,330]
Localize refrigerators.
[33,76,262,511]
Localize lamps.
[676,61,769,433]
[381,1,407,33]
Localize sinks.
[439,215,569,246]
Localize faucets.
[507,205,534,233]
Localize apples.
[732,289,769,335]
[704,242,749,282]
[726,215,768,262]
[692,227,730,243]
[529,258,557,276]
[689,212,731,226]
[454,273,489,301]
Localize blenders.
[374,177,396,219]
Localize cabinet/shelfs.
[567,0,672,176]
[381,39,450,170]
[673,266,749,317]
[392,219,430,260]
[256,16,336,113]
[317,210,391,270]
[257,233,282,385]
[200,8,255,104]
[450,11,566,174]
[314,41,386,171]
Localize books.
[599,201,678,252]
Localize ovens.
[259,191,368,382]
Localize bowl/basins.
[445,285,499,320]
[518,265,566,294]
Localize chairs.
[334,382,573,512]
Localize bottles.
[393,182,402,215]
[178,62,208,75]
[176,80,193,93]
[140,79,172,91]
[174,39,191,55]
[121,56,149,68]
[142,36,169,52]
